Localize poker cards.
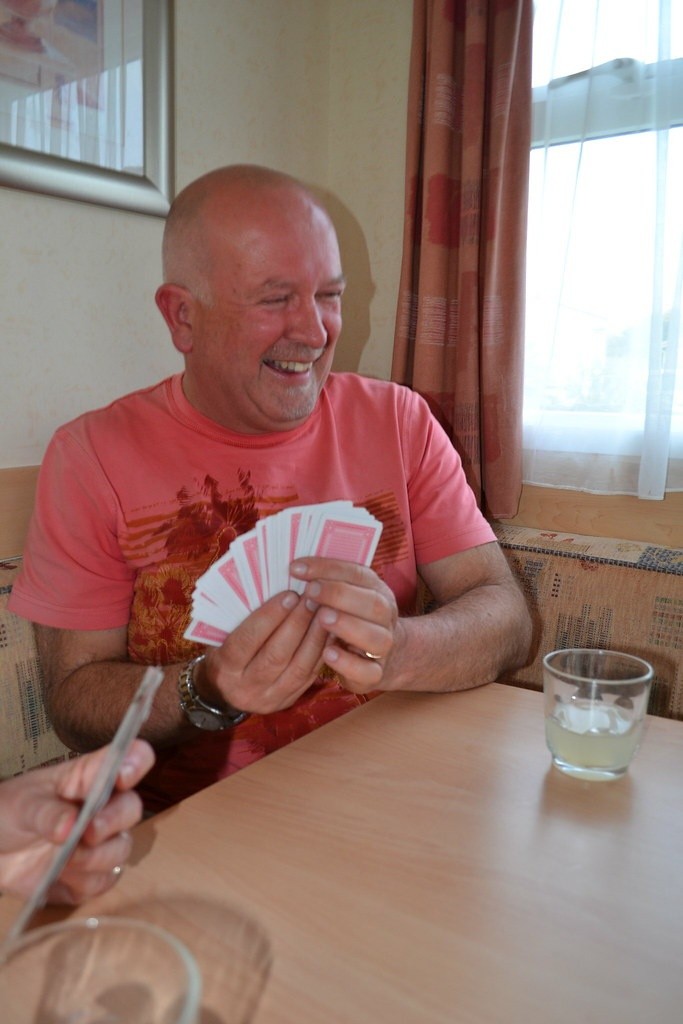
[8,666,165,936]
[184,499,384,646]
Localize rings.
[112,865,124,874]
[363,650,382,660]
[115,830,128,840]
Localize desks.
[0,680,682,1024]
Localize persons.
[0,740,157,906]
[4,164,533,818]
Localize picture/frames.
[0,0,174,217]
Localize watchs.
[177,655,245,731]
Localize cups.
[543,649,654,780]
[0,920,203,1024]
